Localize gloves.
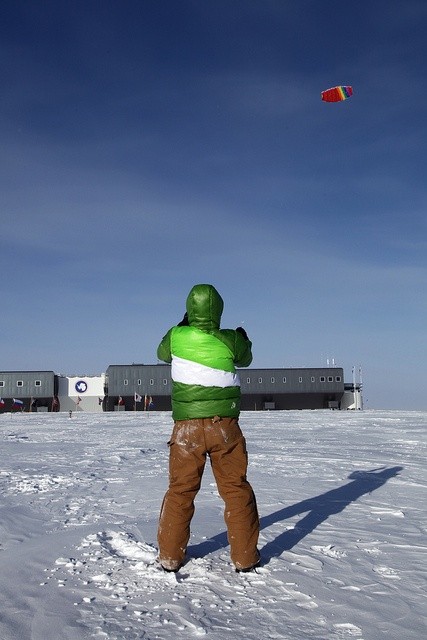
[236,326,249,343]
[176,312,188,326]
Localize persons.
[157,284,261,573]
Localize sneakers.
[235,548,261,570]
[162,547,189,571]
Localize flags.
[118,397,125,406]
[0,397,5,409]
[78,397,81,404]
[31,399,37,407]
[145,395,152,407]
[134,391,142,402]
[11,398,24,411]
[99,398,102,405]
[52,397,59,406]
